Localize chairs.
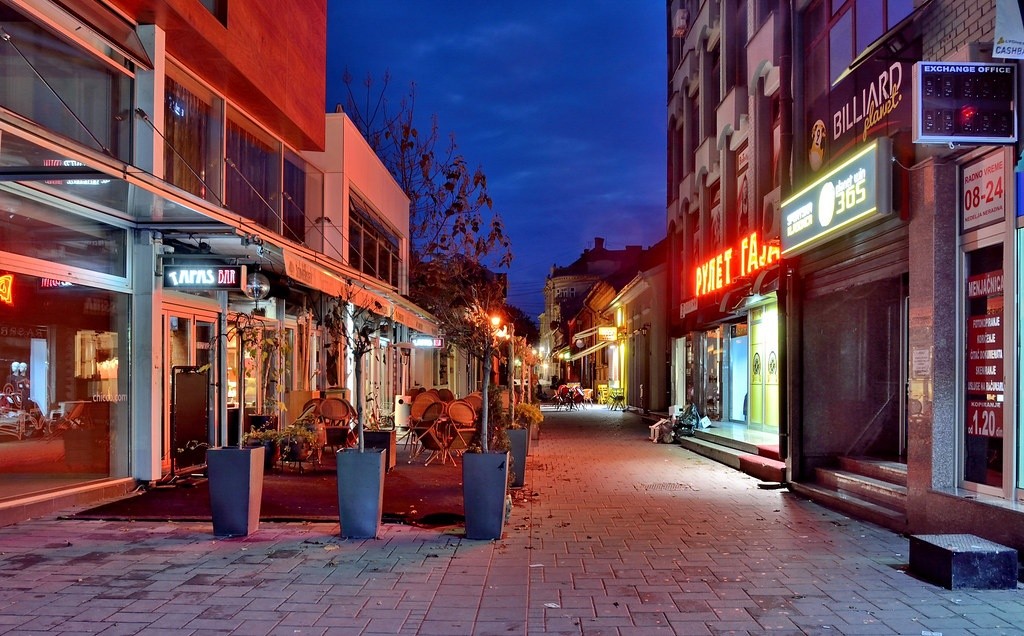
[294,381,518,468]
[553,381,627,412]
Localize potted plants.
[245,425,281,471]
[432,261,511,542]
[312,275,398,540]
[204,311,275,538]
[281,426,317,473]
